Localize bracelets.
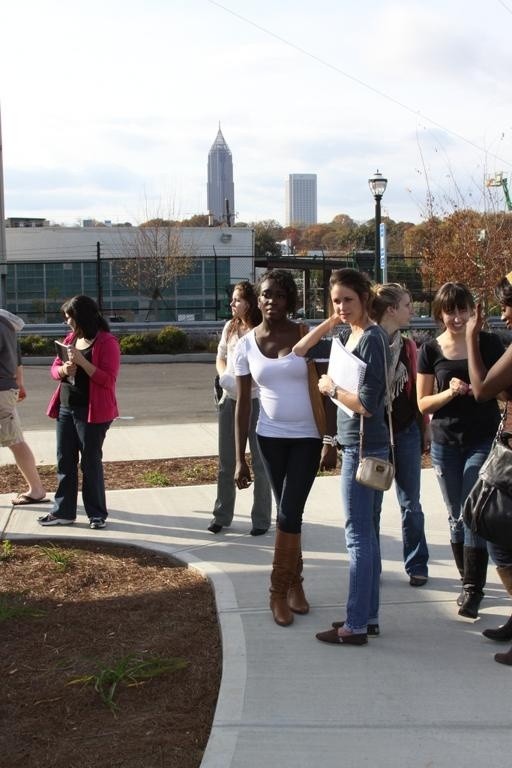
[320,435,339,451]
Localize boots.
[269,528,301,626]
[494,647,512,666]
[483,564,512,641]
[446,539,485,606]
[287,544,310,614]
[458,545,489,619]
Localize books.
[323,339,366,419]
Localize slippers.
[12,495,50,504]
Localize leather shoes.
[208,523,223,533]
[332,621,380,636]
[250,529,267,536]
[410,566,428,586]
[316,629,368,645]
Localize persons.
[315,269,392,647]
[36,293,119,529]
[415,283,507,619]
[365,284,430,587]
[466,272,511,666]
[206,281,274,537]
[1,309,49,506]
[234,269,337,626]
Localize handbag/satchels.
[299,323,328,437]
[462,400,512,540]
[213,337,228,403]
[355,333,395,491]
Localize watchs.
[329,385,338,400]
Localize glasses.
[63,315,71,321]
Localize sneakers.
[88,516,107,529]
[37,512,74,525]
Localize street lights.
[368,170,387,286]
